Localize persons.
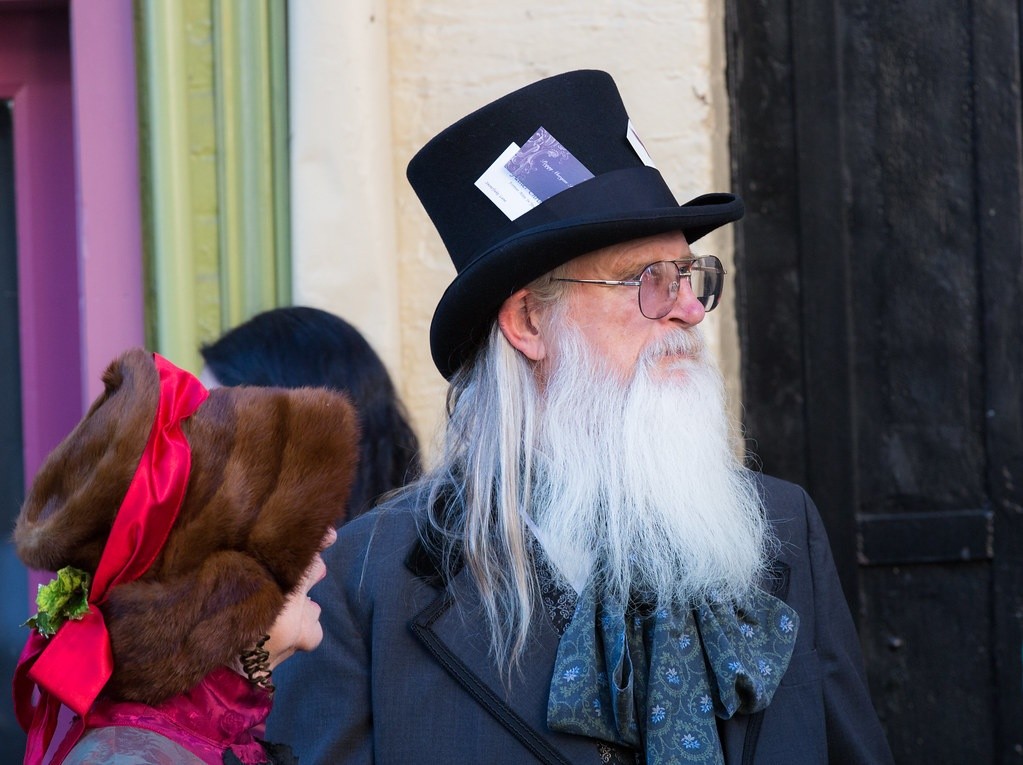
[197,307,425,531]
[11,344,361,765]
[258,67,892,765]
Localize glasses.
[549,254,727,320]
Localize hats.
[11,348,357,765]
[406,69,744,387]
